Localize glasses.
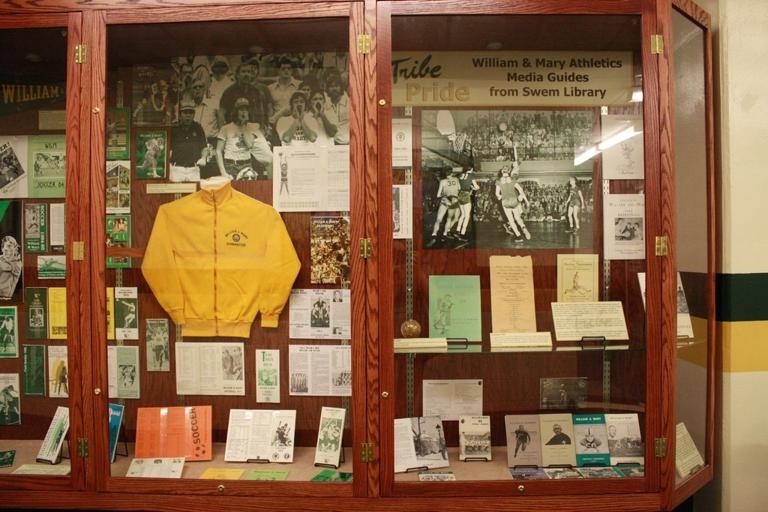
[243,175,256,179]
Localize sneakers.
[504,222,531,242]
[431,231,468,241]
[565,227,579,234]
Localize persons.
[511,423,532,458]
[1,143,67,425]
[422,109,642,241]
[289,216,351,454]
[546,422,573,446]
[579,427,604,449]
[106,50,350,196]
[106,214,170,389]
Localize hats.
[235,98,250,108]
[180,103,195,111]
[212,61,229,73]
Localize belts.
[171,160,195,167]
[226,159,251,165]
